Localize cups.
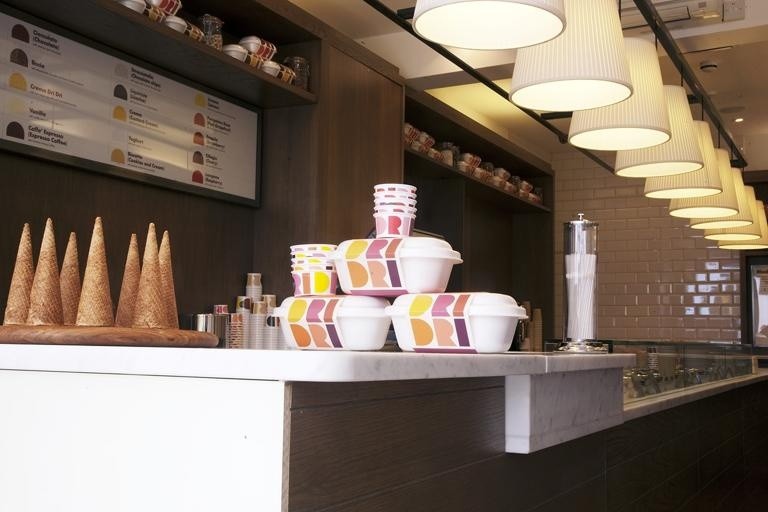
[522,300,543,352]
[647,346,658,370]
[289,244,339,297]
[372,183,419,239]
[214,272,289,348]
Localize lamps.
[414,1,768,253]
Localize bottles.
[480,163,493,176]
[197,14,225,53]
[281,57,310,92]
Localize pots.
[180,312,229,348]
